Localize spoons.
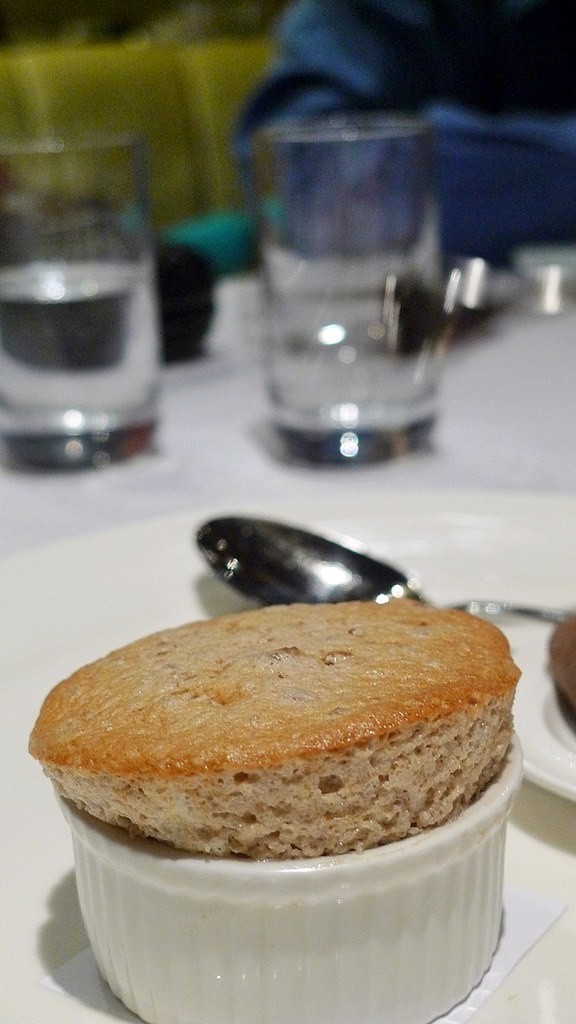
[194,516,570,626]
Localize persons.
[153,0,576,364]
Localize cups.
[55,732,522,1024]
[2,126,161,473]
[256,120,448,465]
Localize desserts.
[25,596,522,860]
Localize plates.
[511,649,576,804]
